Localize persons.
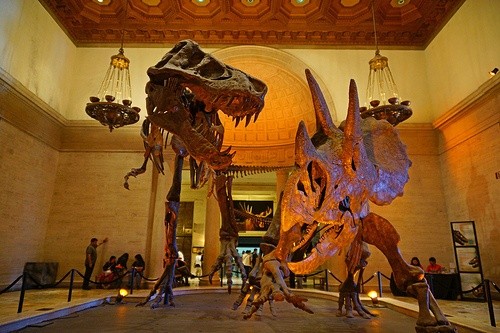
[194,252,202,277]
[410,257,424,270]
[131,254,146,288]
[200,250,204,269]
[175,258,197,286]
[83,238,108,290]
[235,248,259,281]
[99,255,117,289]
[426,257,442,273]
[110,252,129,288]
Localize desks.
[390,273,461,300]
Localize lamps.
[367,291,379,306]
[358,0,413,127]
[115,289,129,304]
[85,0,142,133]
